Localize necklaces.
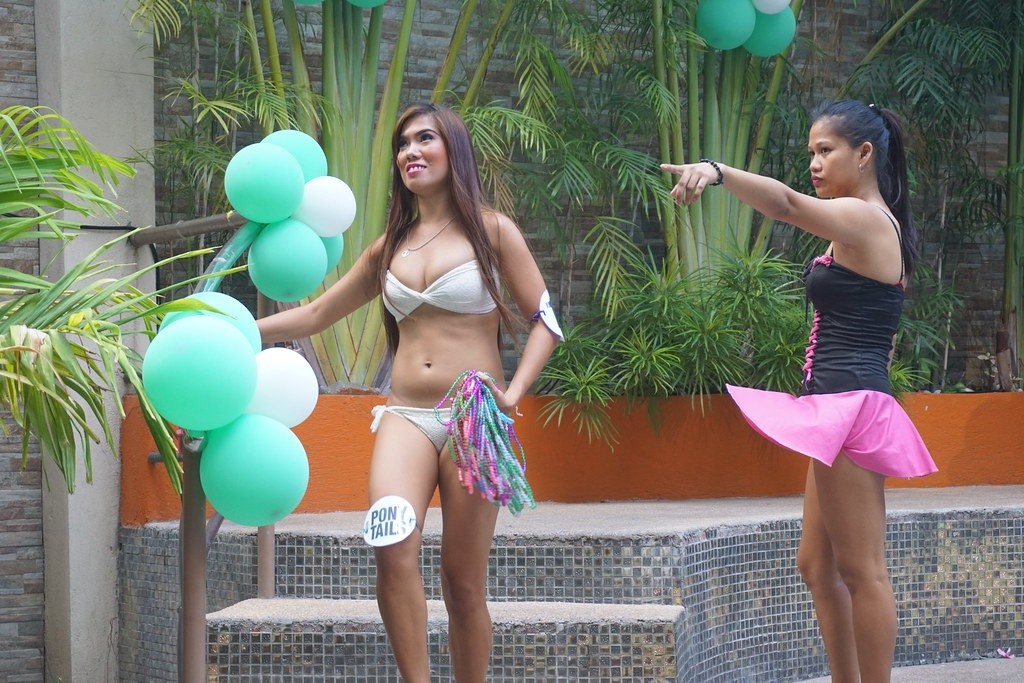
[401,215,456,258]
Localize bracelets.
[698,159,724,187]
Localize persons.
[255,104,564,683]
[659,102,938,683]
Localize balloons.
[224,129,357,303]
[141,292,319,527]
[696,0,796,57]
[291,0,387,8]
